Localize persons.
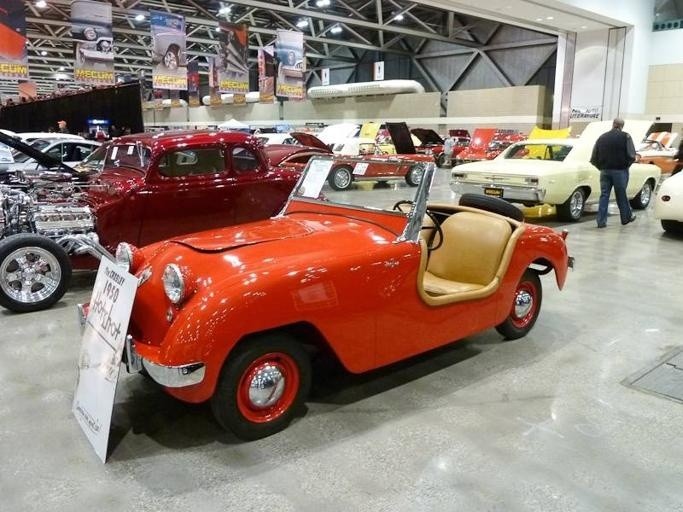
[589,118,636,228]
[57,121,70,134]
[95,125,131,141]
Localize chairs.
[381,204,434,247]
[424,211,512,295]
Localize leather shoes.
[622,215,636,225]
[598,223,607,228]
